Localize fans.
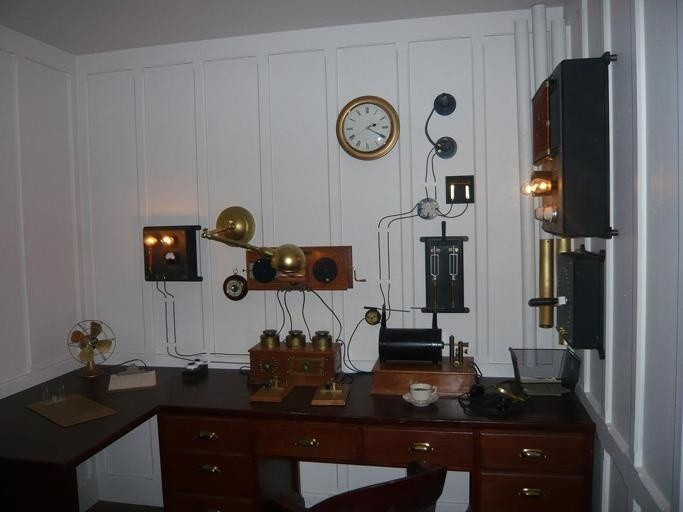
[66,319,117,379]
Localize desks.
[0,360,596,510]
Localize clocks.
[336,94,400,160]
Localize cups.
[410,383,437,401]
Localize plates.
[402,392,439,407]
